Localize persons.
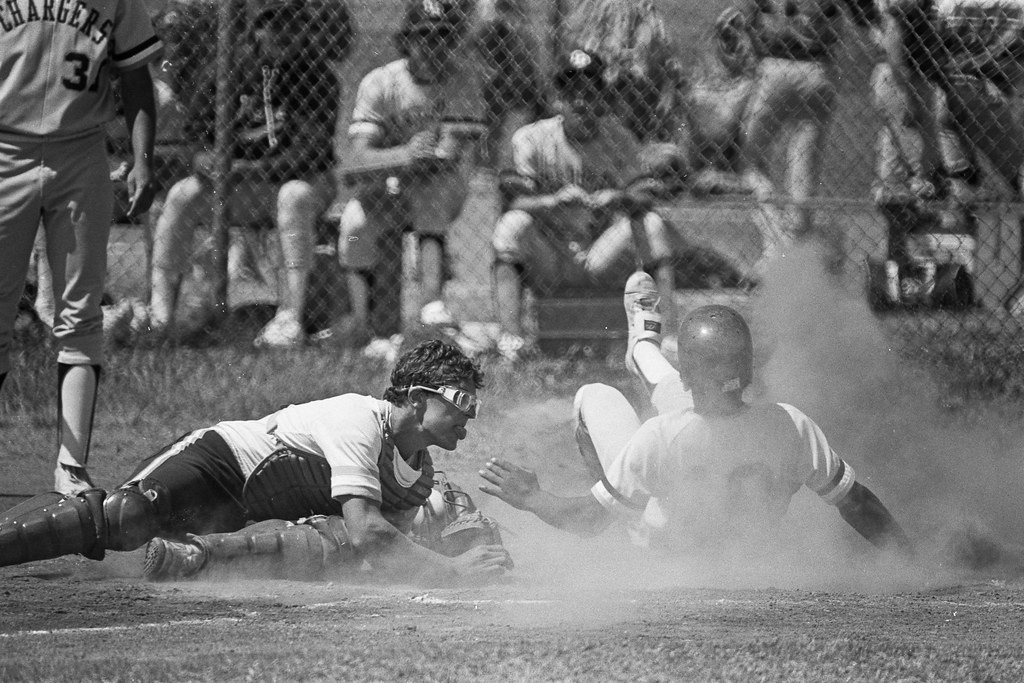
[478,269,920,579]
[0,2,162,501]
[0,342,514,589]
[32,1,1023,359]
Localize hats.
[552,49,606,85]
[394,1,466,49]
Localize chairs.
[523,261,675,360]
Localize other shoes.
[254,309,306,350]
[136,321,176,347]
[309,316,373,349]
[490,334,537,375]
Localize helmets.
[678,307,754,392]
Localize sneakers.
[53,465,95,498]
[625,271,662,379]
[142,538,190,582]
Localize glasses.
[416,385,481,419]
